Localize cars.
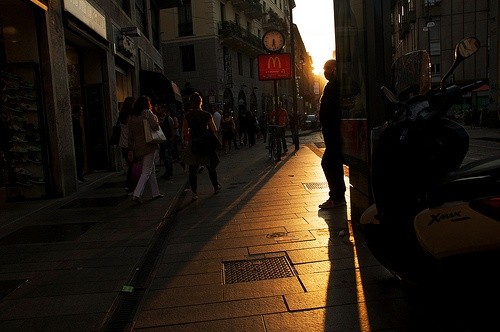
[304,113,320,129]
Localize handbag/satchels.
[111,125,120,146]
[207,112,223,150]
[143,109,166,142]
[118,124,133,148]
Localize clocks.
[262,29,285,53]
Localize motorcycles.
[354,35,500,332]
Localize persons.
[119,97,140,192]
[128,96,165,204]
[319,59,346,210]
[181,91,222,200]
[469,103,500,131]
[72,105,89,184]
[147,99,298,180]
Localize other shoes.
[133,196,144,205]
[193,193,198,200]
[319,199,346,208]
[215,184,222,193]
[153,194,165,200]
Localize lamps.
[122,27,142,38]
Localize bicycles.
[266,125,289,160]
